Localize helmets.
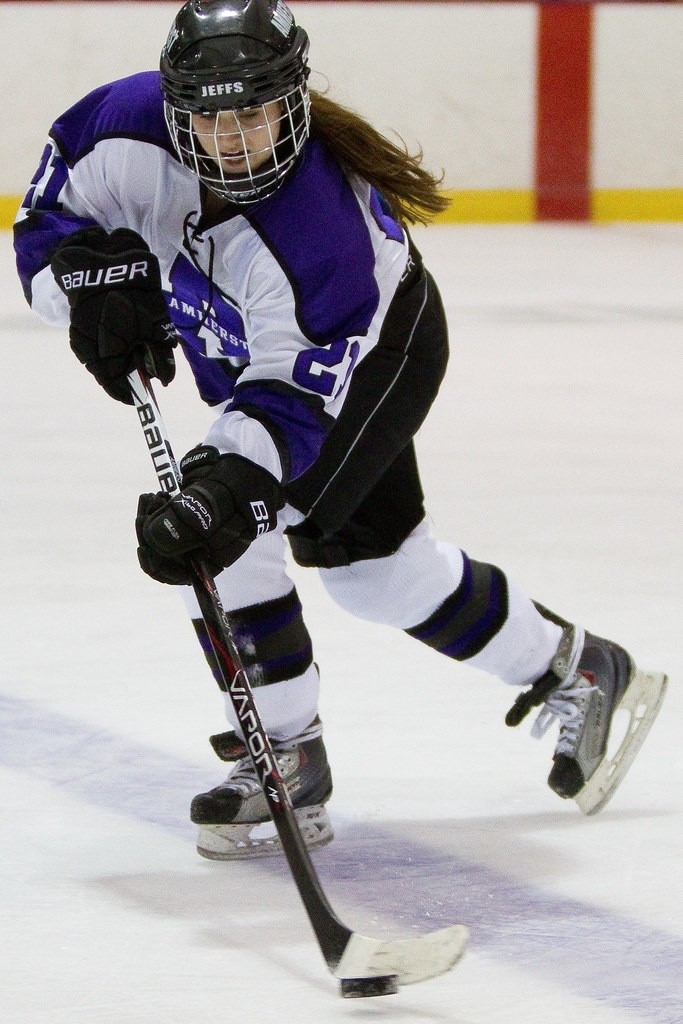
[160,0,312,204]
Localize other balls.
[336,971,404,1001]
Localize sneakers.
[506,599,668,817]
[191,713,334,861]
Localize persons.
[15,2,669,865]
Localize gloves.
[135,444,286,586]
[51,228,178,405]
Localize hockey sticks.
[121,349,475,991]
